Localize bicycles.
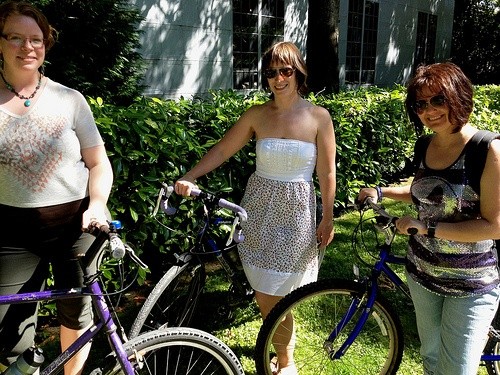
[127,181,256,340]
[0,221,245,375]
[254,189,500,375]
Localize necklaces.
[0,71,41,107]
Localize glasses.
[0,31,49,48]
[409,94,449,115]
[262,67,297,78]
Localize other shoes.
[275,360,298,375]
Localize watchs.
[427,219,437,239]
[375,185,382,202]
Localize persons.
[358,62,500,375]
[174,39,336,375]
[0,0,113,375]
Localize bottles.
[0,347,45,375]
[223,240,245,271]
